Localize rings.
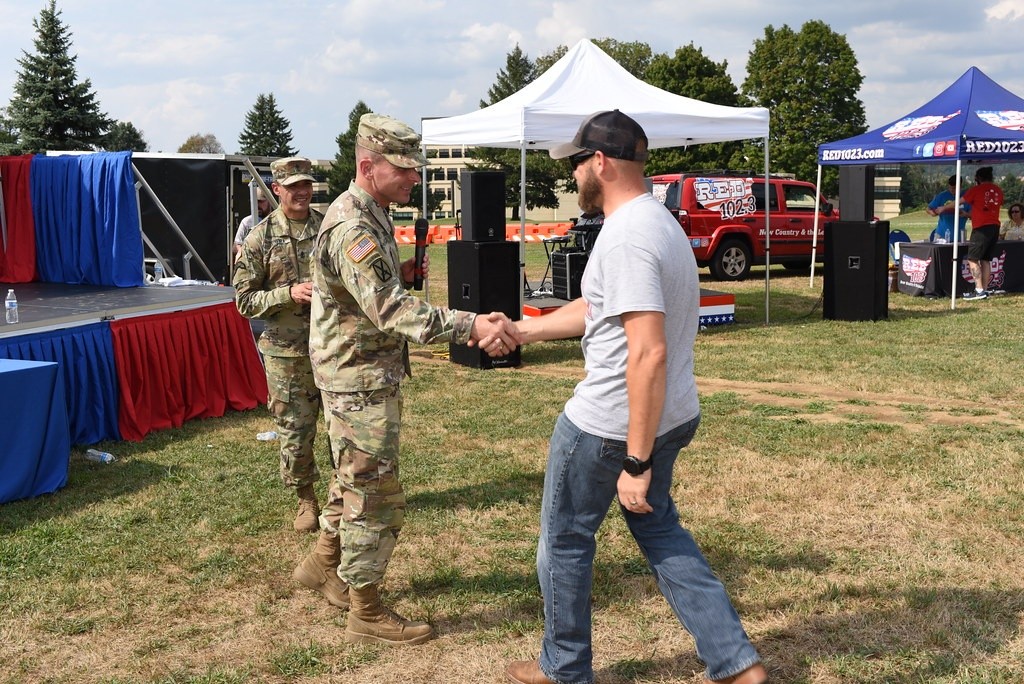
[499,342,505,350]
[630,502,636,504]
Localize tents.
[420,40,771,326]
[808,66,1024,310]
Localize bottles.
[84,449,115,464]
[5,288,19,324]
[257,432,280,440]
[154,260,163,282]
[945,229,950,243]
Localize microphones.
[413,218,430,291]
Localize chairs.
[888,229,911,265]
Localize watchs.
[623,454,653,476]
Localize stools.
[539,238,571,290]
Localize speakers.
[825,220,889,323]
[460,171,507,242]
[447,240,521,370]
[839,164,875,223]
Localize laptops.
[643,178,653,195]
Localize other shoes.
[963,289,987,301]
[984,288,995,298]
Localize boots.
[293,483,320,531]
[292,532,350,610]
[345,586,434,648]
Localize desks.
[0,358,72,505]
[897,240,1024,297]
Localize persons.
[465,107,772,684]
[926,165,1024,301]
[232,110,525,645]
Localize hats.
[976,166,993,182]
[948,174,963,186]
[549,108,650,161]
[355,113,432,169]
[270,157,317,186]
[256,187,271,200]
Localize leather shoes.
[704,663,769,684]
[505,659,555,684]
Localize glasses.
[569,152,595,171]
[1011,210,1020,214]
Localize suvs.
[651,174,839,280]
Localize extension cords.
[533,290,553,296]
